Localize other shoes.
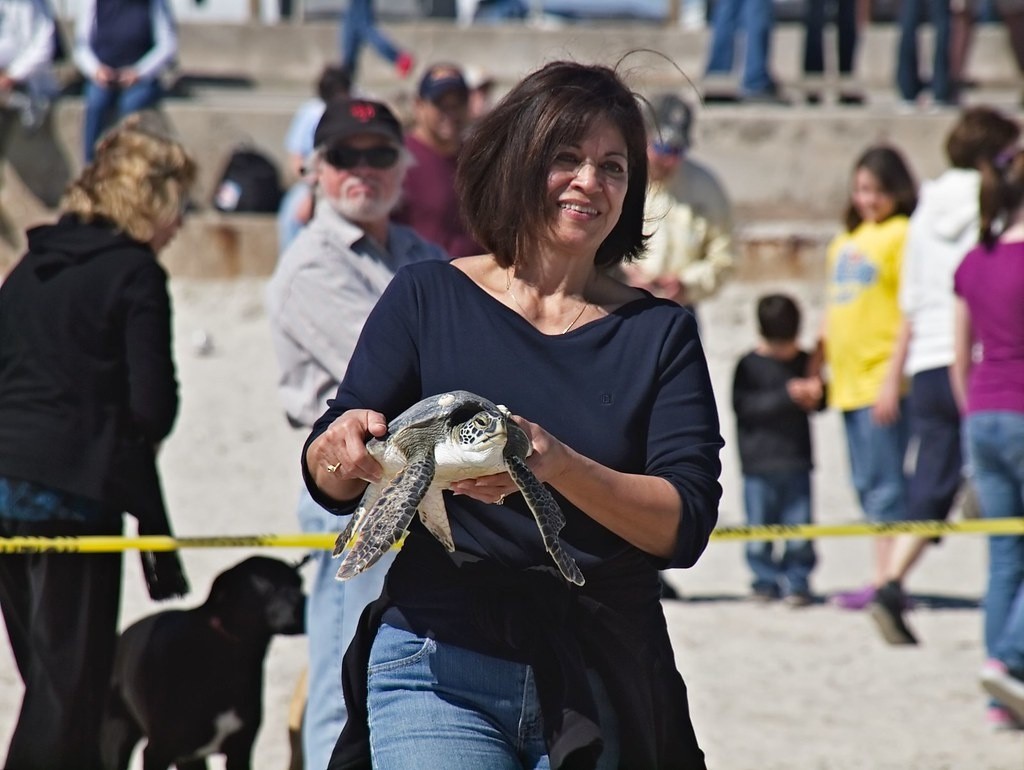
[870,590,916,644]
[832,583,875,607]
[787,590,813,605]
[751,582,778,602]
[980,655,1024,729]
[397,54,412,76]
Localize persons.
[707,0,973,104]
[821,144,916,612]
[0,0,57,96]
[299,61,727,770]
[732,295,828,608]
[951,136,1024,732]
[868,109,1020,642]
[610,95,740,302]
[278,67,348,252]
[0,110,200,770]
[268,98,447,770]
[339,0,415,78]
[74,0,179,168]
[389,65,491,258]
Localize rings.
[328,463,340,473]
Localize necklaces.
[506,266,599,334]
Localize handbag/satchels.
[211,150,278,213]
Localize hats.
[645,96,691,146]
[420,67,466,99]
[315,100,402,148]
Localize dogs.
[98,554,307,770]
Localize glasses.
[326,145,399,168]
[652,142,682,157]
